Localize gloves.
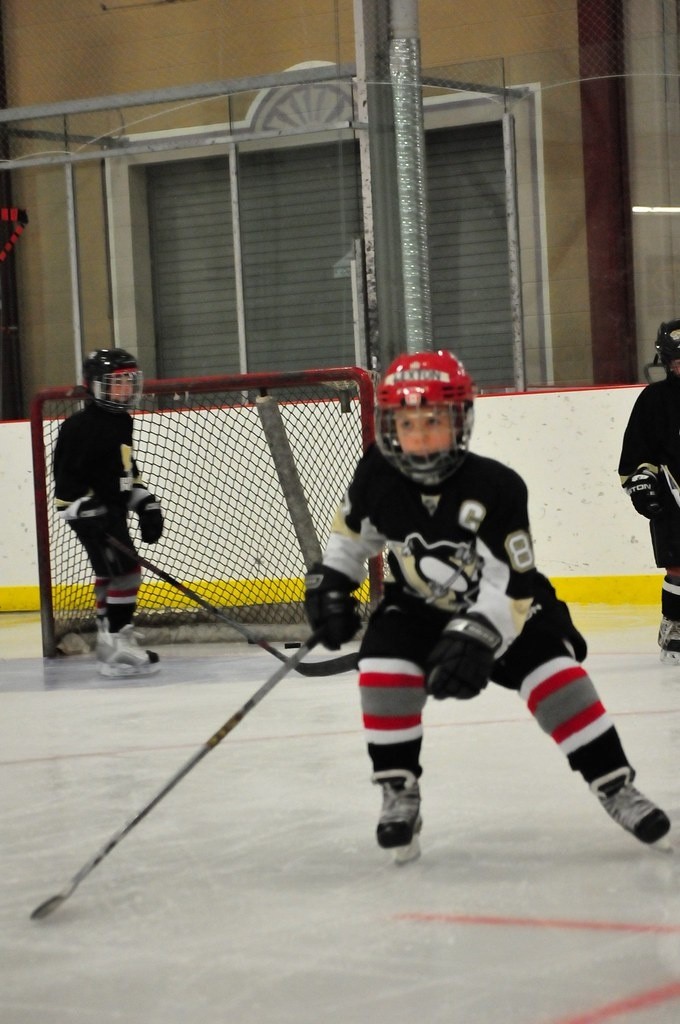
[627,476,660,519]
[76,502,108,545]
[132,495,163,542]
[428,612,504,701]
[302,563,363,652]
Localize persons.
[50,347,163,677]
[618,320,679,663]
[304,352,672,864]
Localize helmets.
[655,319,680,376]
[375,350,477,485]
[82,348,141,411]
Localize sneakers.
[370,765,422,866]
[95,625,161,676]
[590,768,672,853]
[657,615,680,665]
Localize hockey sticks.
[105,532,360,679]
[28,631,322,922]
